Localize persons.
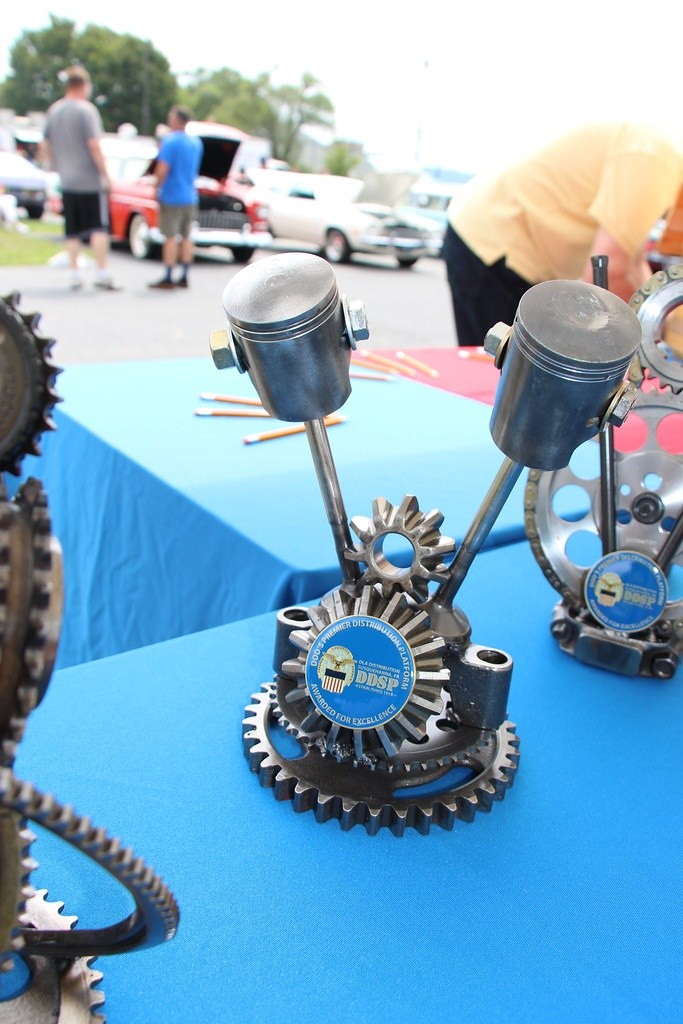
[147,103,205,289]
[442,116,682,348]
[44,63,123,292]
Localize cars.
[0,153,45,218]
[239,169,426,268]
[47,123,274,260]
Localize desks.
[3,288,682,1024]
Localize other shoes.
[150,281,173,289]
[175,281,187,288]
[71,280,83,291]
[94,278,120,290]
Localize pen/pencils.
[194,391,348,446]
